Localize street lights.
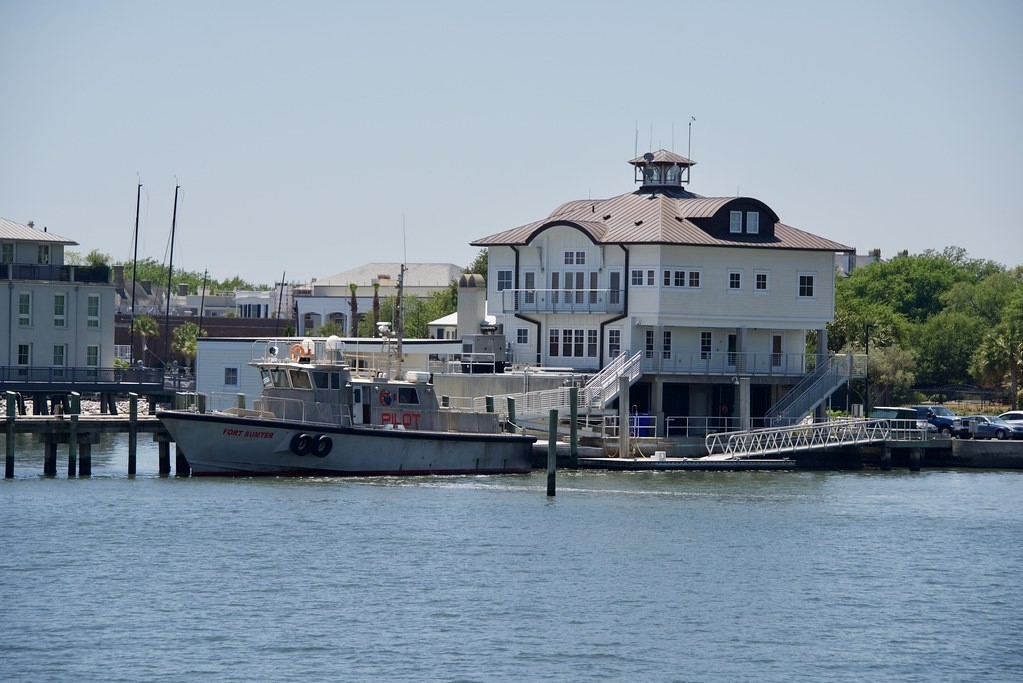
[865,324,879,424]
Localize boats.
[154,263,539,477]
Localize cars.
[961,415,1018,440]
[997,410,1023,440]
[916,421,939,434]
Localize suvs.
[911,405,963,440]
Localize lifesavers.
[315,434,333,459]
[380,390,393,406]
[291,344,304,361]
[290,432,315,457]
